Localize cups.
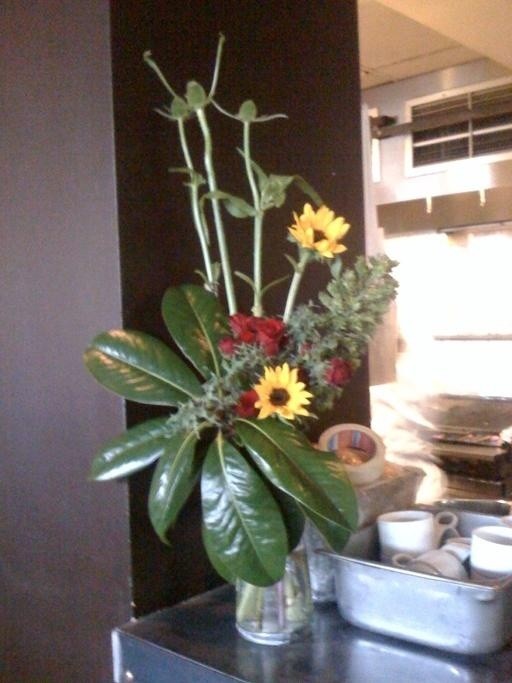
[377,508,512,580]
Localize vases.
[232,497,316,648]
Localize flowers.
[80,29,402,588]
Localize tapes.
[317,421,387,487]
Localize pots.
[401,391,511,436]
